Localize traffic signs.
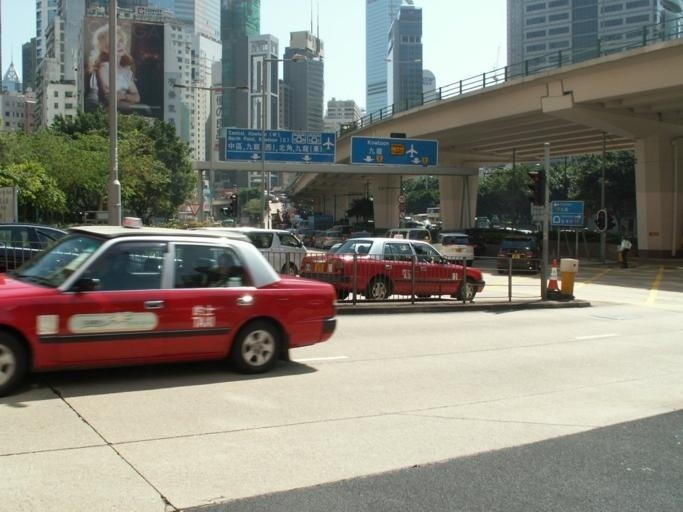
[229,130,334,163]
[351,138,437,167]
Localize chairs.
[191,258,241,282]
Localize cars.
[385,227,543,278]
[302,238,484,299]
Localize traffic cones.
[546,257,560,292]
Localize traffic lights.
[596,210,608,232]
[527,169,544,206]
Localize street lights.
[257,49,307,228]
[172,83,250,215]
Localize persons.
[209,252,238,288]
[618,235,632,269]
[87,23,141,115]
